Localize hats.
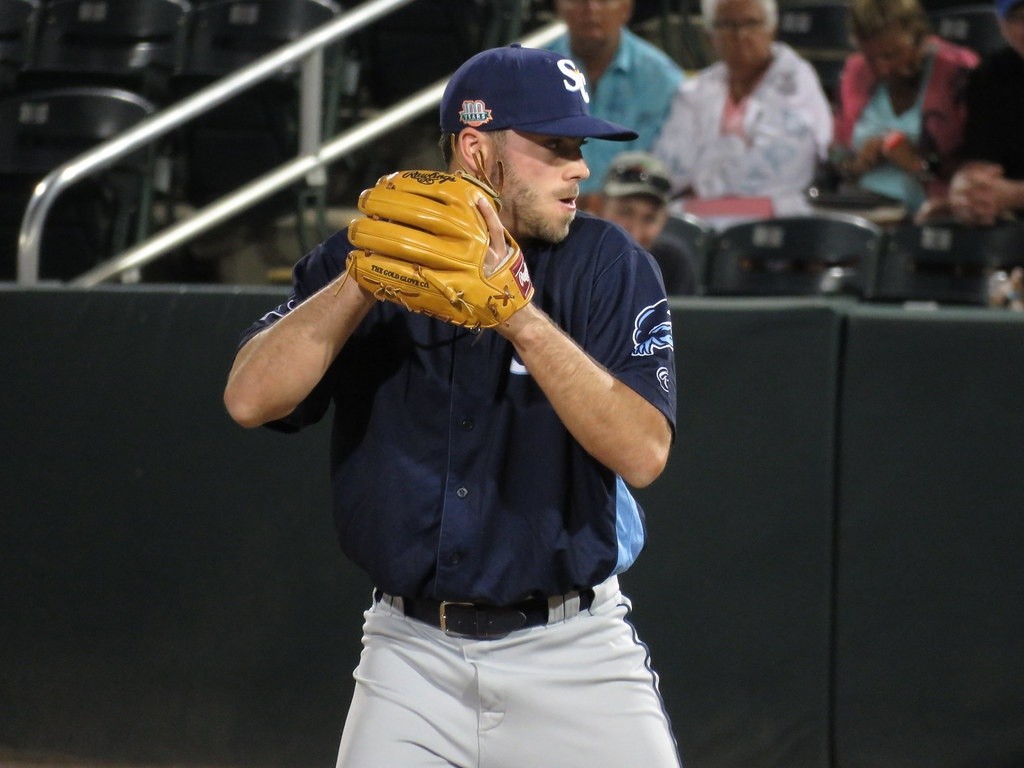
[604,150,673,204]
[440,44,639,141]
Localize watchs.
[912,159,931,175]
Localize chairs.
[24,1,188,97]
[178,75,296,222]
[359,0,506,119]
[651,211,706,293]
[0,90,164,276]
[634,0,1003,96]
[177,1,337,91]
[714,217,880,299]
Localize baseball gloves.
[347,171,536,327]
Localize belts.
[375,586,593,641]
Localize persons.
[642,0,833,227]
[821,1,980,225]
[922,1,1024,308]
[579,153,701,303]
[223,40,691,768]
[512,1,687,199]
